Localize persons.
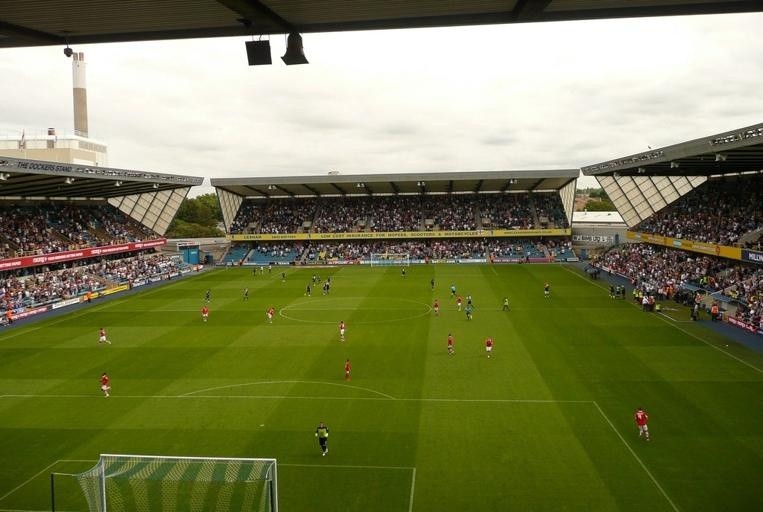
[337,320,346,342]
[254,266,272,275]
[589,172,763,336]
[431,279,435,290]
[634,409,652,442]
[202,306,210,324]
[100,375,114,398]
[434,299,439,312]
[244,287,249,301]
[345,359,351,383]
[227,192,572,265]
[545,284,550,298]
[450,284,478,320]
[266,306,275,323]
[203,287,213,303]
[484,335,494,360]
[306,274,330,297]
[447,334,455,357]
[503,298,510,311]
[401,266,406,278]
[282,271,287,278]
[0,201,202,326]
[98,327,110,344]
[314,421,329,454]
